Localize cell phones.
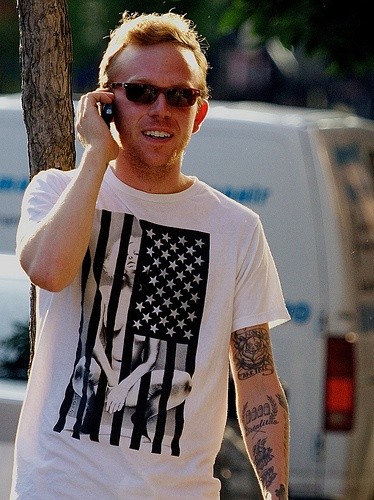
[100,101,115,128]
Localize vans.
[0,91,374,498]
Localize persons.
[10,10,292,500]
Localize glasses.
[107,82,203,107]
